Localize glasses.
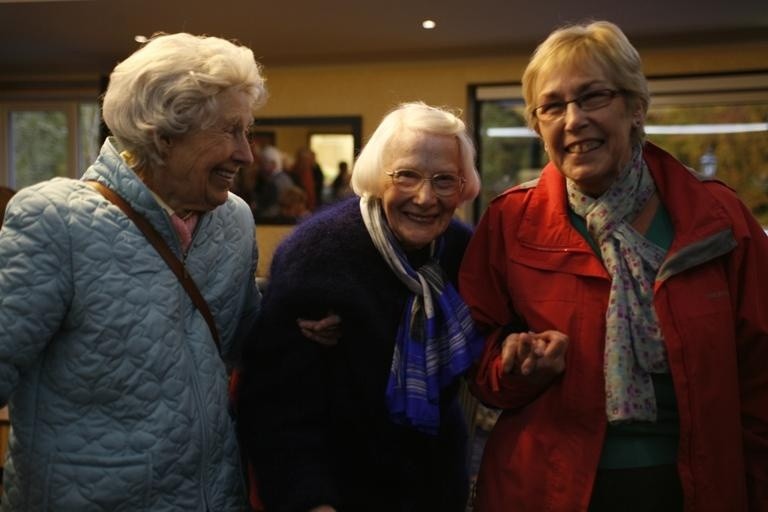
[383,168,468,199]
[532,87,631,124]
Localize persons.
[0,30,347,510]
[236,98,546,512]
[455,21,768,511]
[240,131,353,226]
